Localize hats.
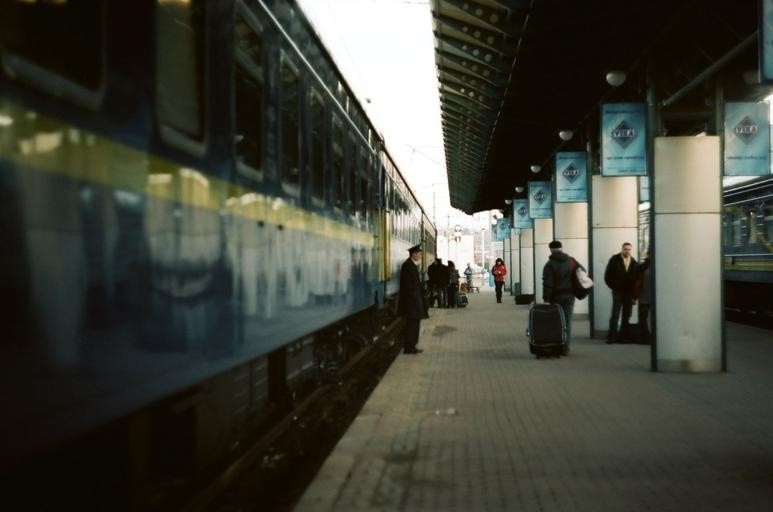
[550,241,562,248]
[408,244,422,253]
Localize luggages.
[525,302,568,359]
[454,288,468,307]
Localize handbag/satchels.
[571,266,594,300]
[631,271,643,298]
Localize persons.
[426,255,474,310]
[631,249,653,346]
[543,241,586,357]
[395,243,429,354]
[492,257,507,303]
[346,244,378,310]
[604,242,639,340]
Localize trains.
[1,0,439,511]
[642,172,773,323]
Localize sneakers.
[438,304,454,308]
[402,346,424,354]
